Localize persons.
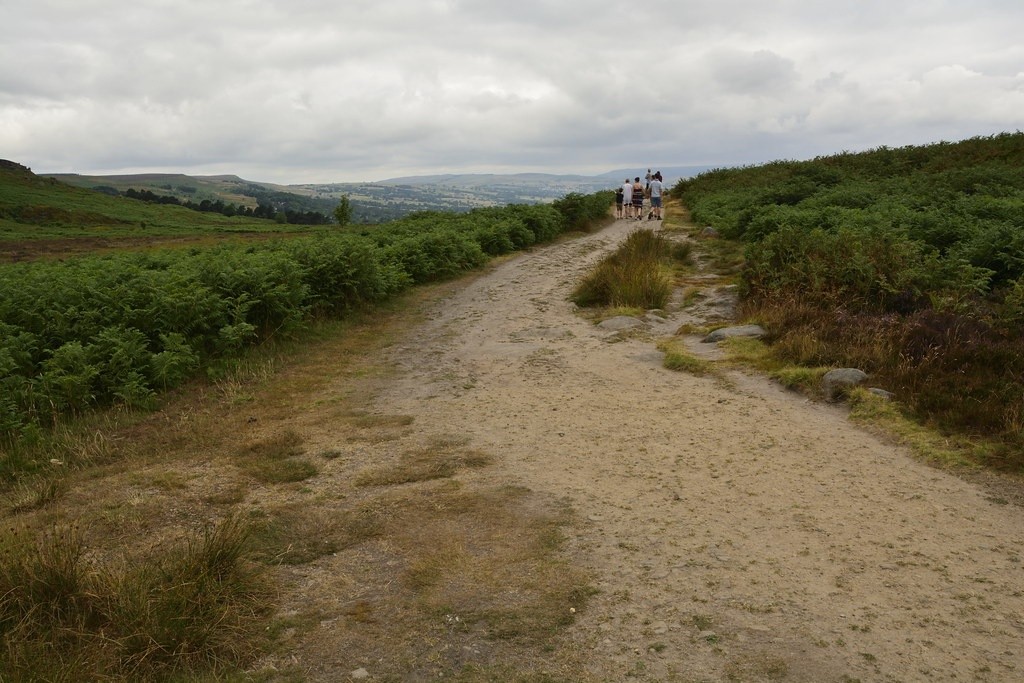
[622,178,633,219]
[657,171,662,182]
[631,177,644,220]
[648,173,662,220]
[615,189,624,219]
[645,169,651,189]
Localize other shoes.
[617,217,619,221]
[648,211,652,220]
[638,215,642,221]
[625,216,628,218]
[657,216,663,220]
[629,214,633,218]
[620,217,624,219]
[634,217,637,221]
[654,214,657,219]
